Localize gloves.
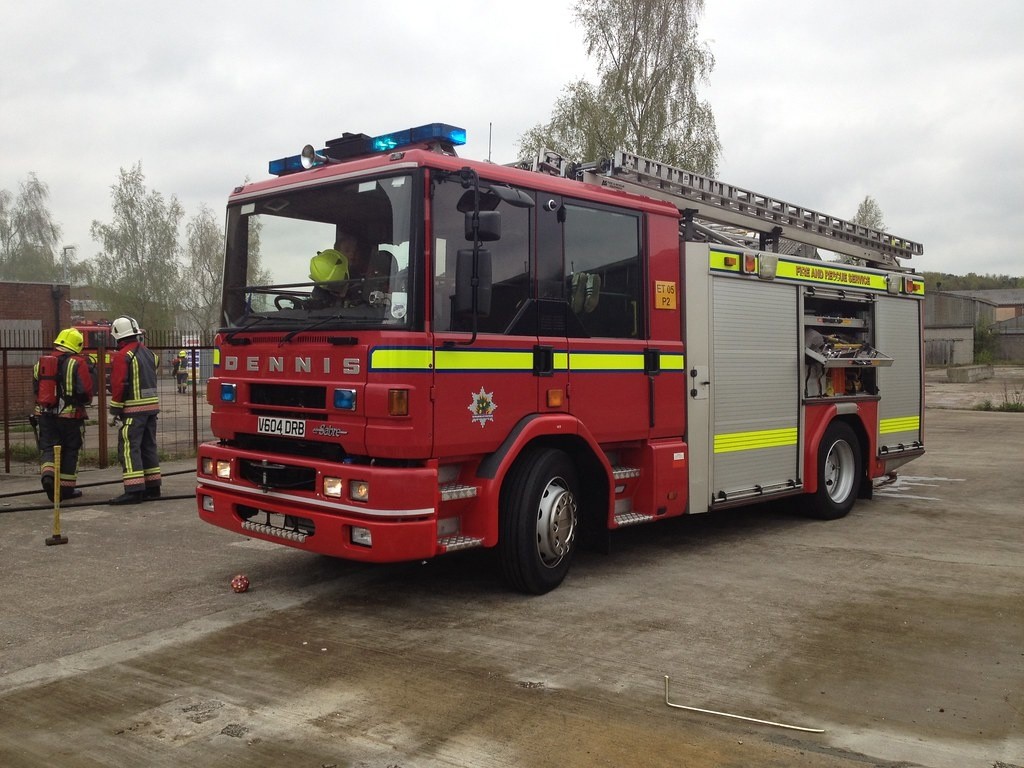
[107,414,117,427]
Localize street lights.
[63,244,76,283]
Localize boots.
[41,466,64,503]
[108,473,162,505]
[59,478,82,499]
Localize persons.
[33,328,93,502]
[301,235,396,309]
[108,317,162,504]
[172,350,188,394]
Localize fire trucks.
[69,318,149,395]
[197,122,927,593]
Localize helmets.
[54,328,83,354]
[179,351,186,356]
[110,315,142,339]
[308,249,350,292]
